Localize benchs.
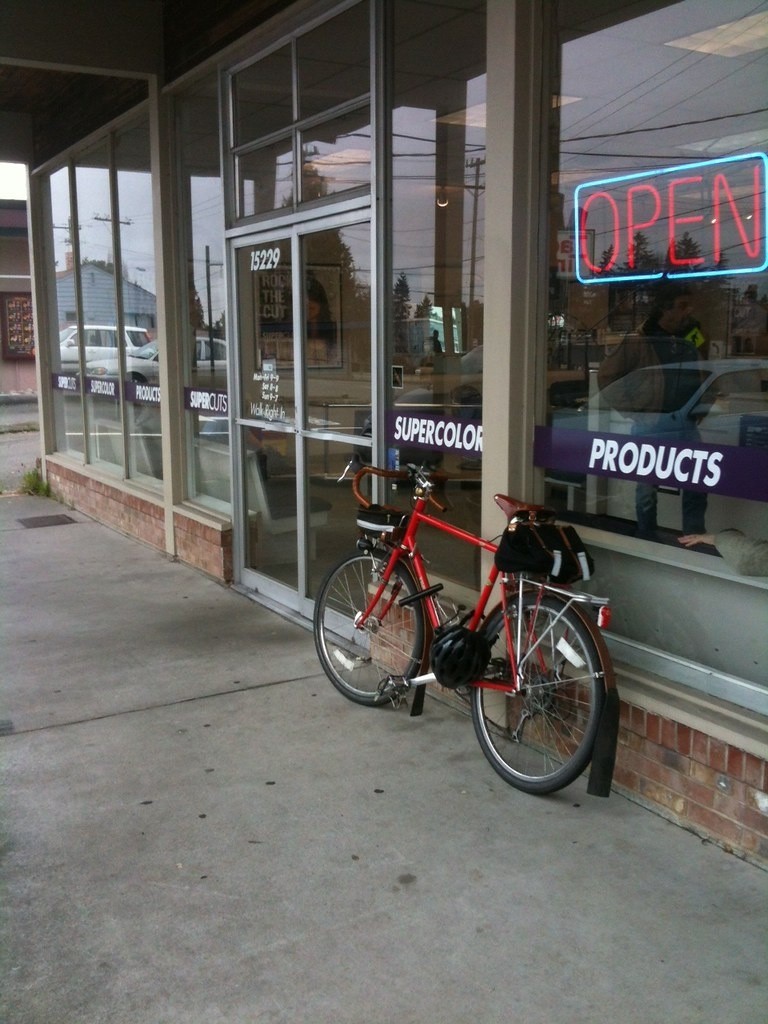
[91,421,332,562]
[531,459,767,686]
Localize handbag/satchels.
[494,513,596,585]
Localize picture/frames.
[250,261,342,367]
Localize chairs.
[354,385,482,490]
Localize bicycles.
[311,452,622,799]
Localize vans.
[59,323,152,375]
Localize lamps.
[437,187,449,207]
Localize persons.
[278,278,335,338]
[598,286,719,535]
[678,528,768,577]
[430,330,443,353]
[202,420,285,507]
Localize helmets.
[429,626,491,690]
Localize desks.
[299,403,481,482]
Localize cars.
[547,356,768,505]
[76,335,229,406]
[360,333,720,463]
